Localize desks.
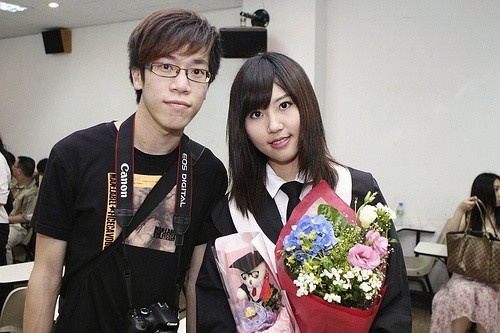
[0,262,67,284]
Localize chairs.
[13,226,34,263]
[0,287,27,333]
[397,226,436,314]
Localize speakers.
[42,29,72,54]
[219,26,267,58]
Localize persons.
[430,173,500,333]
[196,52,412,333]
[23,10,229,333]
[0,138,49,316]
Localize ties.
[280,182,304,225]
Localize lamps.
[219,9,270,59]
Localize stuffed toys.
[236,261,279,311]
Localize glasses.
[142,62,214,83]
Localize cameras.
[126,302,179,333]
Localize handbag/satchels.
[446,199,500,284]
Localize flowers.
[278,191,396,309]
